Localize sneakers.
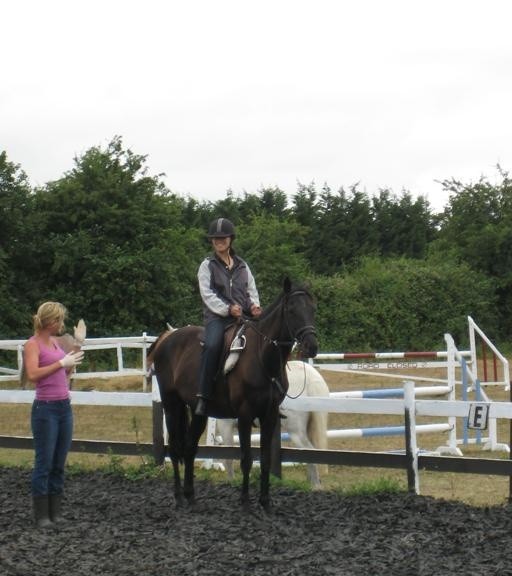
[194,397,208,416]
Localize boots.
[32,490,71,528]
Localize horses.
[164,320,332,493]
[152,276,320,513]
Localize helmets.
[207,218,236,238]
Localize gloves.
[59,350,84,368]
[73,319,87,348]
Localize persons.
[19,300,90,529]
[190,217,263,418]
